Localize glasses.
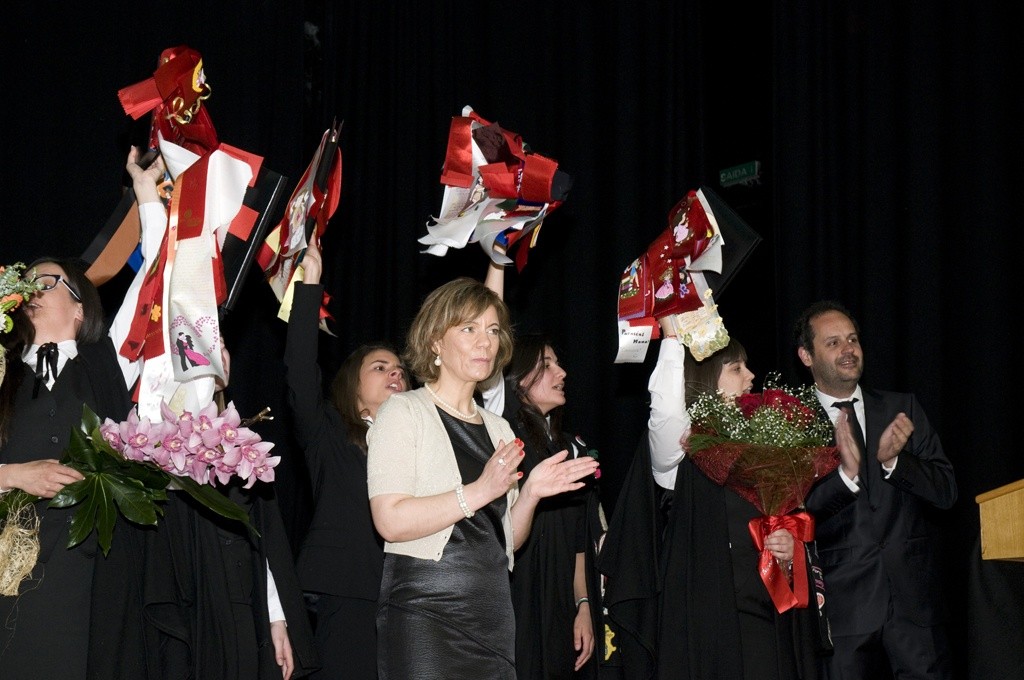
[24,273,82,300]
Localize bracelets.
[660,335,676,338]
[574,597,591,607]
[454,484,474,519]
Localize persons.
[0,254,105,680]
[472,245,624,680]
[283,232,410,680]
[77,142,319,680]
[790,305,951,679]
[366,278,600,680]
[596,314,834,680]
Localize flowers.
[0,399,281,557]
[587,448,602,480]
[0,261,43,333]
[679,370,841,613]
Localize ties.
[831,398,868,493]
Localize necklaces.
[423,381,478,419]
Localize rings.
[498,458,507,467]
